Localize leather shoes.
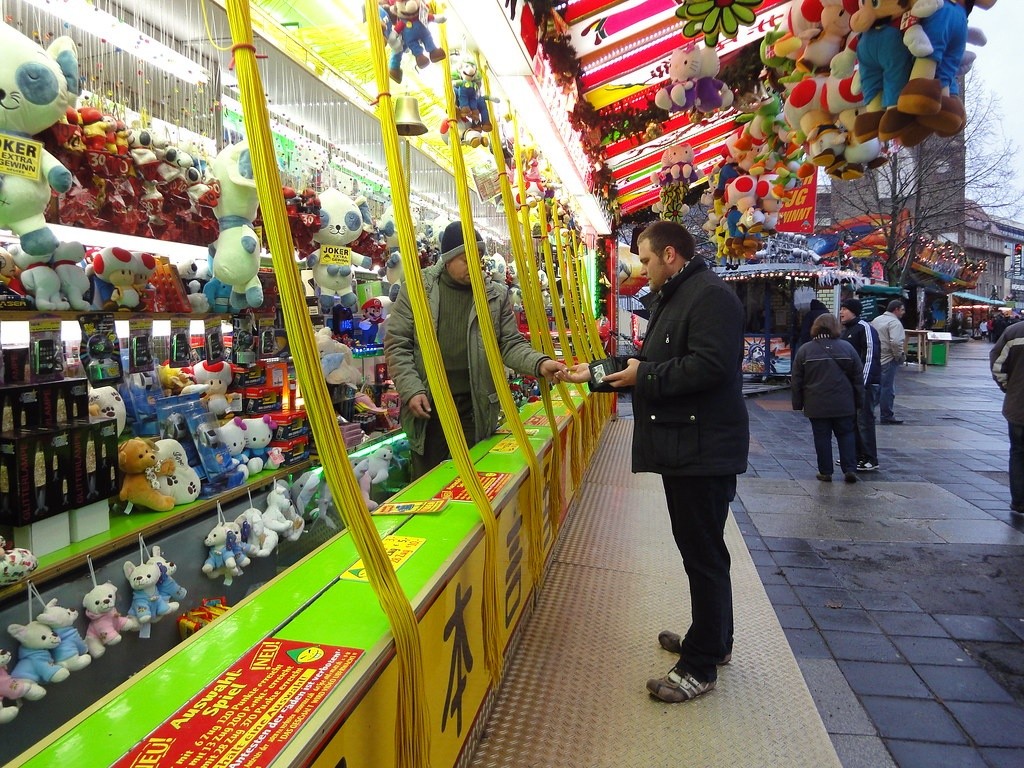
[881,416,904,424]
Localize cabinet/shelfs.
[0,220,569,608]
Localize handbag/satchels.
[855,382,864,409]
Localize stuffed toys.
[654,43,734,112]
[376,0,447,84]
[650,144,705,184]
[201,439,412,586]
[481,252,551,312]
[701,0,997,270]
[0,19,446,511]
[508,145,582,238]
[0,546,190,725]
[440,57,501,148]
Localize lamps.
[755,231,824,265]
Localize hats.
[840,298,863,317]
[441,221,486,263]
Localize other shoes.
[844,472,857,483]
[816,471,832,482]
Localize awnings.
[855,284,911,297]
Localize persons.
[595,373,603,383]
[977,313,1021,343]
[555,221,750,704]
[790,313,866,484]
[989,321,1024,514]
[802,299,829,343]
[868,299,906,425]
[384,221,570,482]
[836,298,880,472]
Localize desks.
[903,328,929,373]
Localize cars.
[166,412,186,441]
[138,372,153,390]
[195,422,218,449]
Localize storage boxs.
[12,511,70,559]
[69,498,111,543]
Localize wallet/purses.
[588,356,647,392]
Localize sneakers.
[835,459,841,466]
[646,662,717,703]
[856,462,880,471]
[658,630,732,666]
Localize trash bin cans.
[928,332,952,365]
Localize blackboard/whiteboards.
[860,296,877,322]
